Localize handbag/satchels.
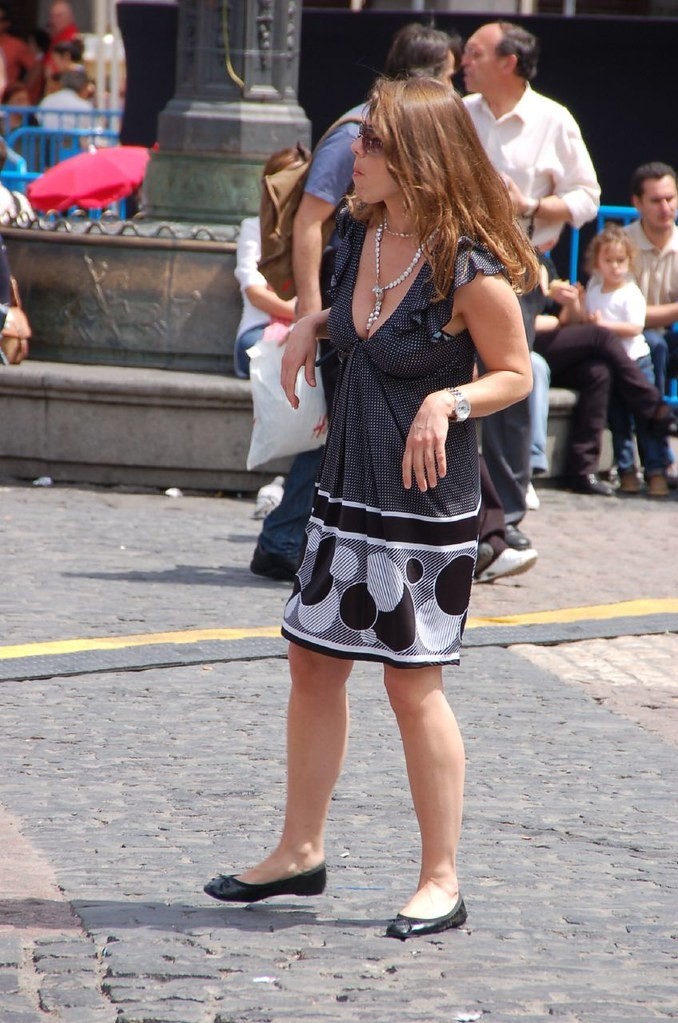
[0,278,32,364]
[245,336,329,471]
[257,117,364,302]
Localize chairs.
[568,206,678,430]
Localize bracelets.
[521,198,540,220]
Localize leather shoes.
[503,523,530,550]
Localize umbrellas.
[28,144,152,212]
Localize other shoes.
[251,543,295,581]
[621,469,639,493]
[477,548,539,582]
[525,482,540,510]
[474,541,493,575]
[647,475,670,496]
[386,892,467,938]
[565,468,613,496]
[203,859,327,902]
[649,404,678,438]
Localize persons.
[0,0,112,365]
[203,75,536,940]
[233,21,603,583]
[573,221,673,496]
[530,233,672,495]
[586,162,678,480]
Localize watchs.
[443,387,472,422]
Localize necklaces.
[383,212,420,239]
[366,222,441,329]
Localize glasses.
[357,121,385,155]
